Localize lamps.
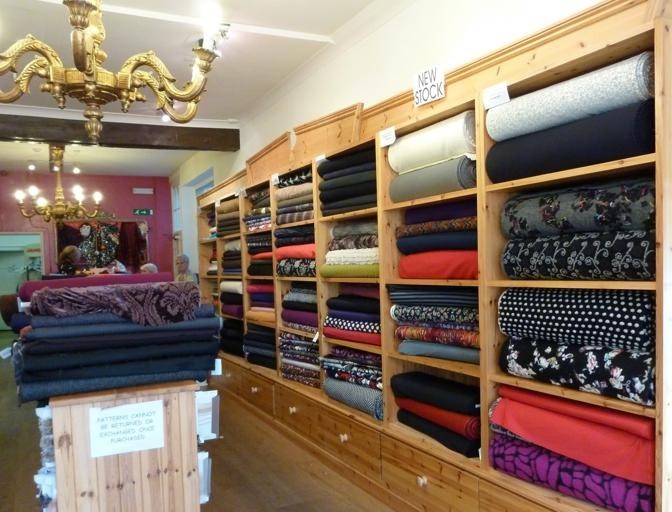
[18,147,101,231]
[0,0,222,145]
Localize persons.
[174,254,198,284]
[140,262,158,274]
[60,244,81,263]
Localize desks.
[48,379,200,512]
